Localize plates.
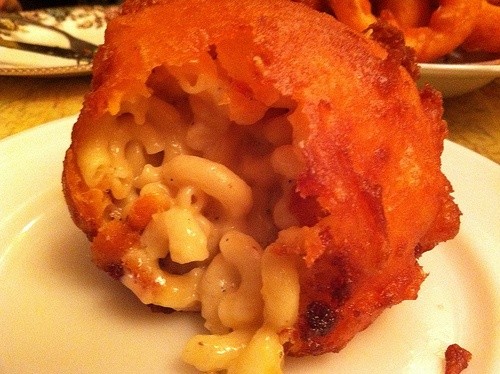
[413,52,500,99]
[0,6,122,76]
[0,112,500,374]
